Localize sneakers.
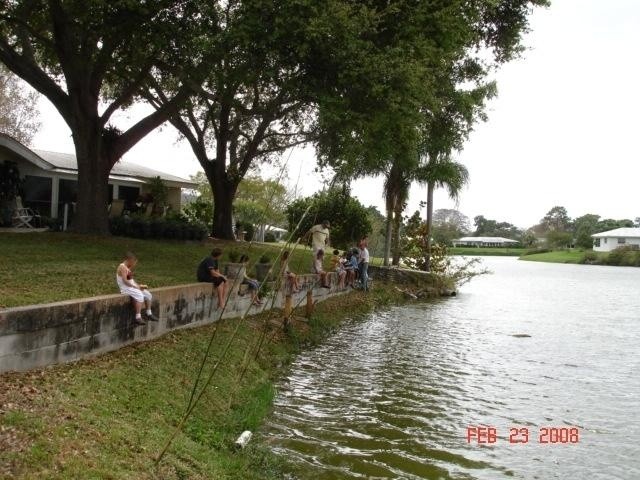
[144,314,159,321]
[134,319,146,325]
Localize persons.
[197,248,228,308]
[303,220,330,273]
[314,239,371,291]
[280,251,301,293]
[117,251,160,325]
[238,255,263,305]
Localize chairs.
[12,196,34,228]
